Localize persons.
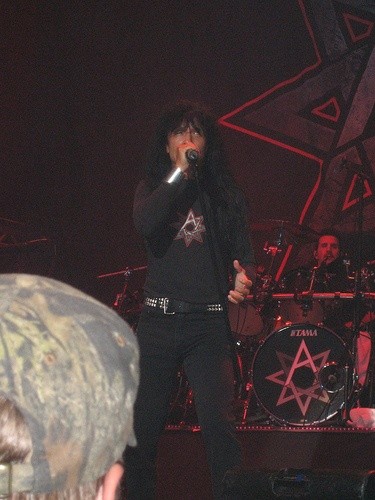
[292,228,375,389]
[122,103,258,500]
[0,271,140,500]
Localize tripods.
[315,175,364,428]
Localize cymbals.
[241,218,320,246]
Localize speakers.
[219,467,375,500]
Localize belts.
[145,298,227,315]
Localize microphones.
[185,149,199,161]
[338,157,375,183]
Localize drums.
[225,263,271,337]
[250,322,357,426]
[273,297,325,333]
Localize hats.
[0,272,140,493]
